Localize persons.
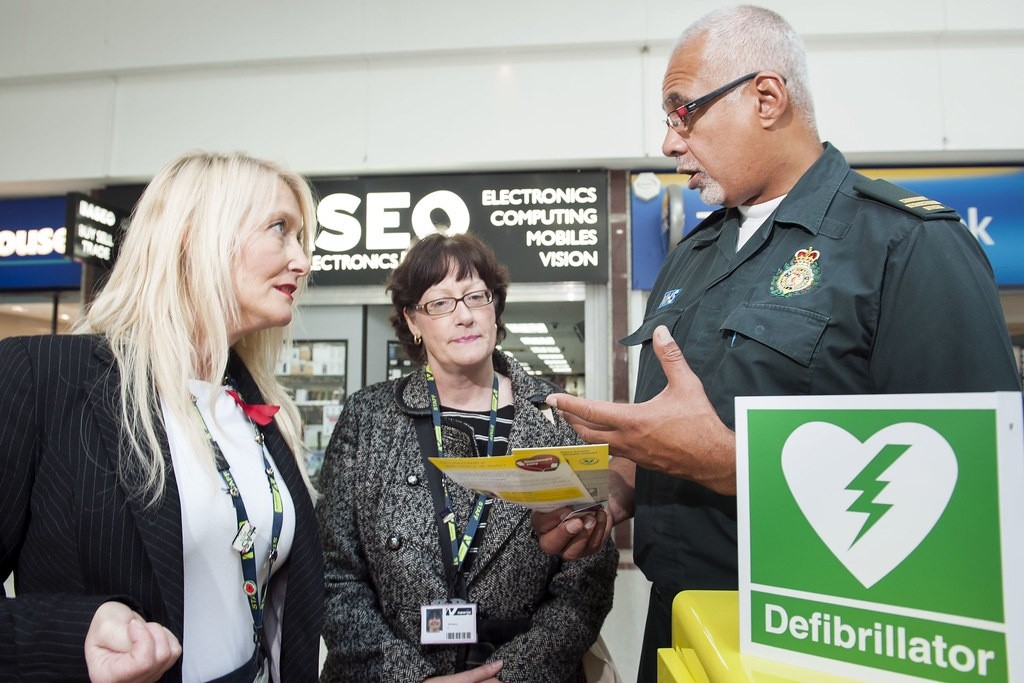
[0,152,319,683]
[551,3,1024,682]
[319,236,619,682]
[427,610,442,631]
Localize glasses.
[411,290,495,316]
[663,71,788,132]
